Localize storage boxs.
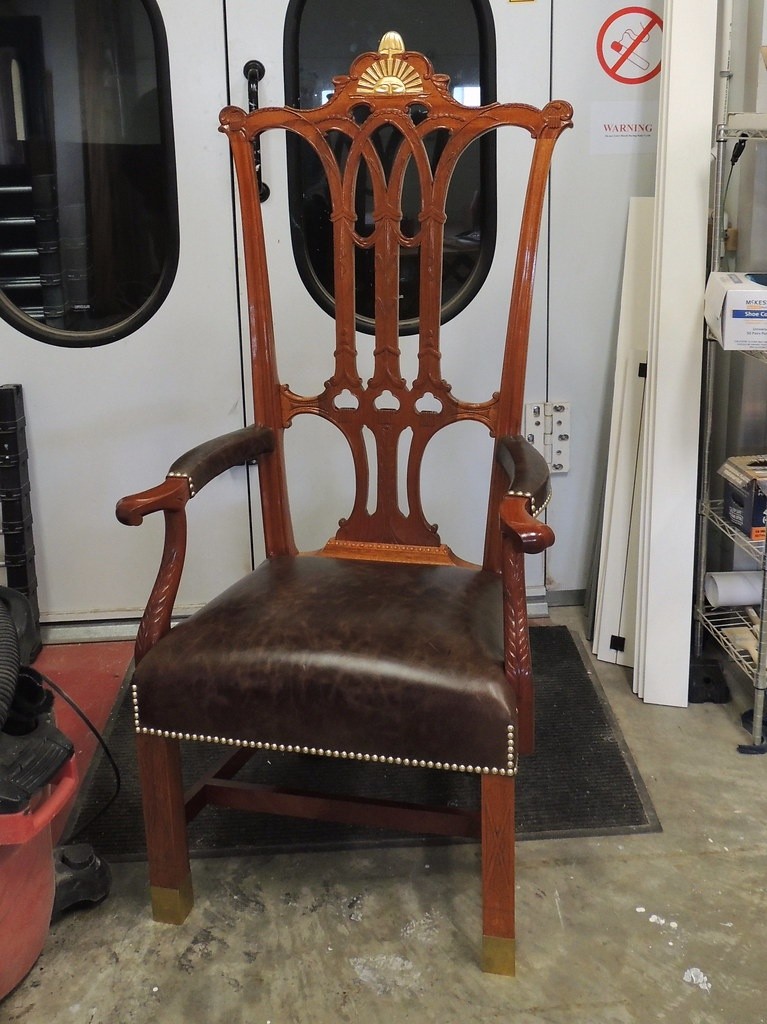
[704,272,767,350]
[715,453,767,539]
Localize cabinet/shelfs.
[691,0,767,753]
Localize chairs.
[115,32,576,940]
